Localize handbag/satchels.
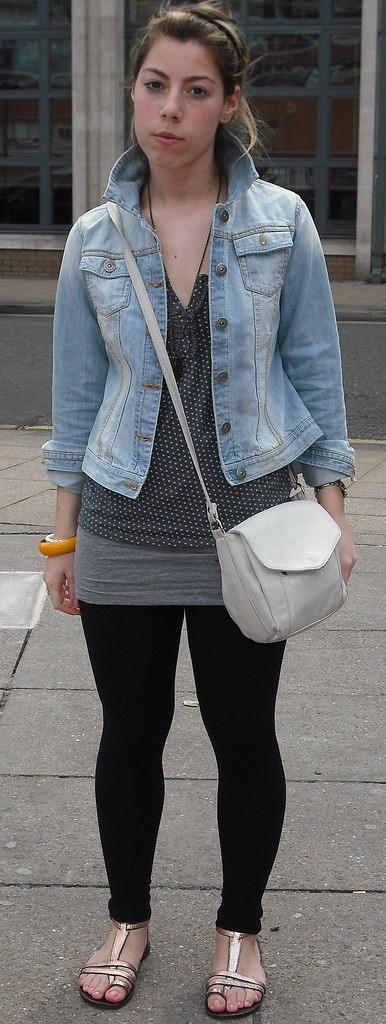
[213,498,348,643]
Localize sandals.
[204,926,266,1019]
[79,918,150,1008]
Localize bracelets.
[39,533,76,556]
[314,479,349,503]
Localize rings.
[46,587,50,596]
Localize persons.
[40,4,358,1014]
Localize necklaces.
[146,169,224,313]
